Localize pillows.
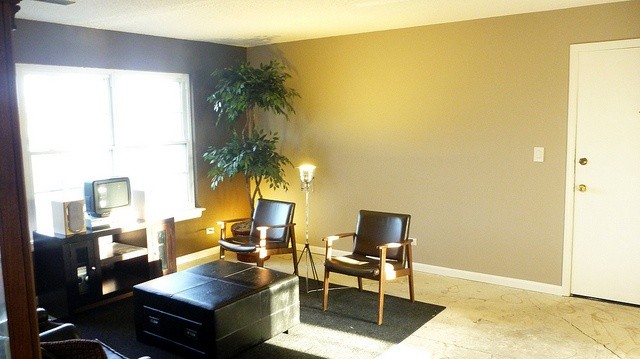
[39,338,107,359]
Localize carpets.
[50,276,447,359]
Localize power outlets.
[206,227,215,234]
[532,147,545,163]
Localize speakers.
[52,198,87,235]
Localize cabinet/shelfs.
[32,210,177,316]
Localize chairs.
[218,198,298,277]
[322,210,415,325]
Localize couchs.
[39,321,151,359]
[0,307,76,359]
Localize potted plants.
[200,62,302,263]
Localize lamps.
[291,163,321,300]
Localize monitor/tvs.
[84,177,132,218]
